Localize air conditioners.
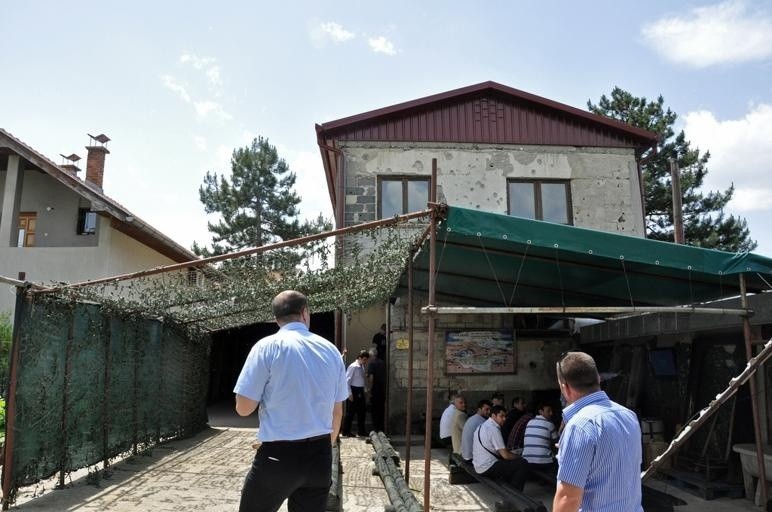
[187,270,204,288]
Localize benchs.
[511,446,689,512]
[447,438,547,512]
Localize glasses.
[557,348,581,387]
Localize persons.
[551,350,647,512]
[341,321,387,436]
[231,289,351,512]
[437,388,566,494]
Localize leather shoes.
[342,432,355,437]
[357,431,370,436]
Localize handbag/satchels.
[498,453,528,467]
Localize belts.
[291,434,331,442]
